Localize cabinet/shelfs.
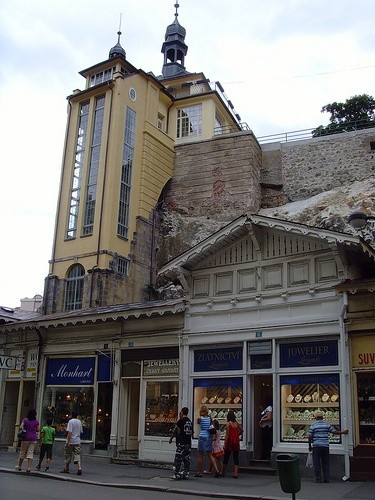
[357,396,375,445]
[282,401,340,443]
[198,402,243,440]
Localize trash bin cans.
[275,452,302,495]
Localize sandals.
[60,469,70,473]
[215,470,222,477]
[194,472,203,477]
[77,470,82,475]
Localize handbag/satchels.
[17,426,27,438]
[305,450,314,468]
[208,417,217,434]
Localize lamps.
[309,288,319,296]
[230,298,239,305]
[281,291,289,299]
[255,295,264,302]
[207,301,215,308]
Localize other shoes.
[312,478,323,483]
[204,470,212,474]
[14,465,22,471]
[45,466,50,472]
[27,468,31,472]
[324,480,331,483]
[231,474,238,479]
[36,464,41,469]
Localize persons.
[258,404,273,458]
[221,411,243,478]
[15,408,40,472]
[169,408,192,479]
[308,410,349,483]
[36,419,56,472]
[195,406,222,477]
[61,411,84,475]
[205,420,224,475]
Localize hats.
[315,412,323,417]
